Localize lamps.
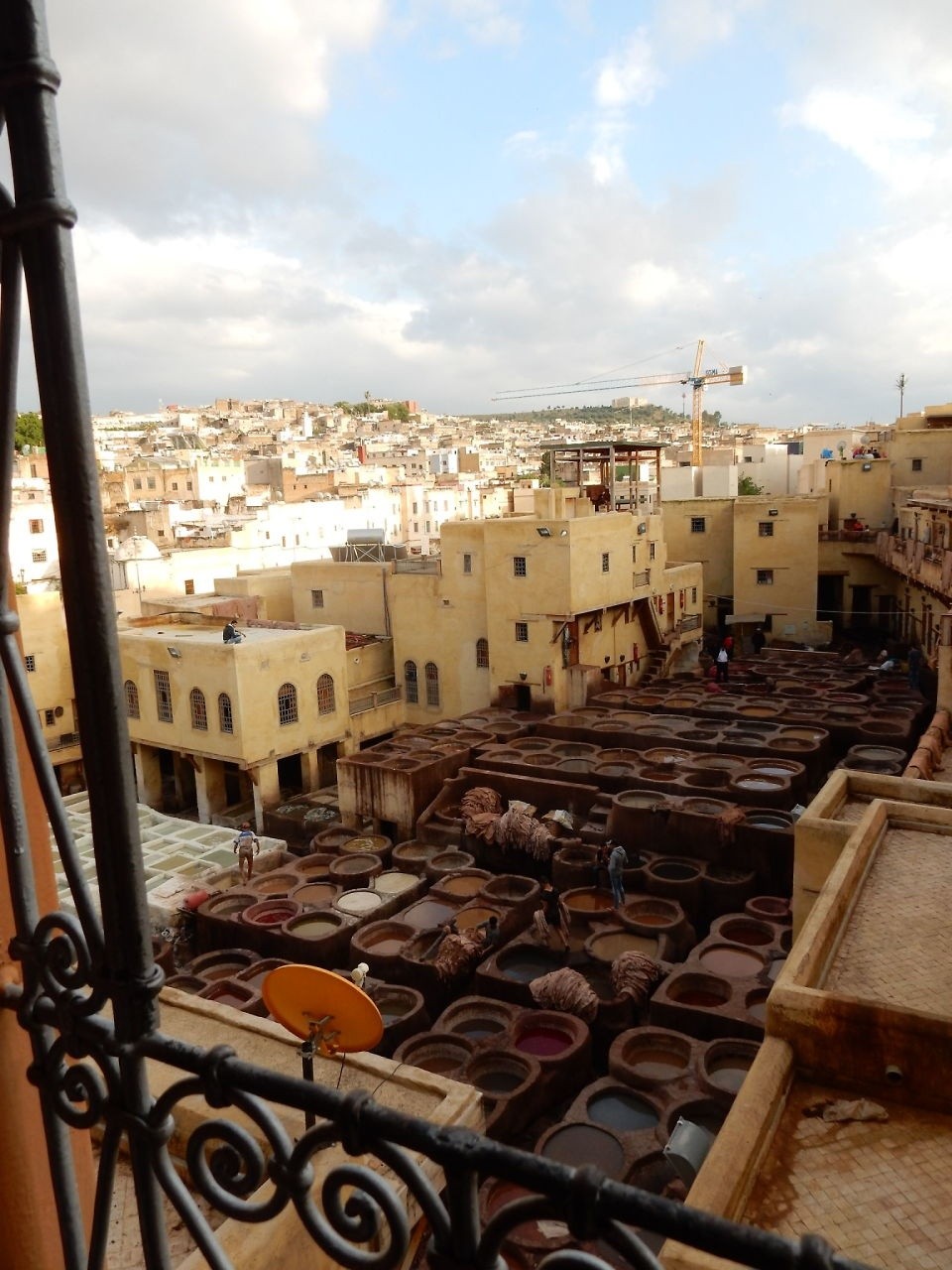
[769,508,778,516]
[862,463,871,471]
[638,522,646,534]
[168,645,181,659]
[538,527,551,537]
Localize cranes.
[491,338,749,466]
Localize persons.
[852,445,881,459]
[233,822,260,877]
[595,839,627,909]
[223,620,246,644]
[877,642,921,690]
[539,876,573,953]
[712,628,767,682]
[474,916,500,953]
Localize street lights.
[896,373,911,418]
[682,392,687,422]
